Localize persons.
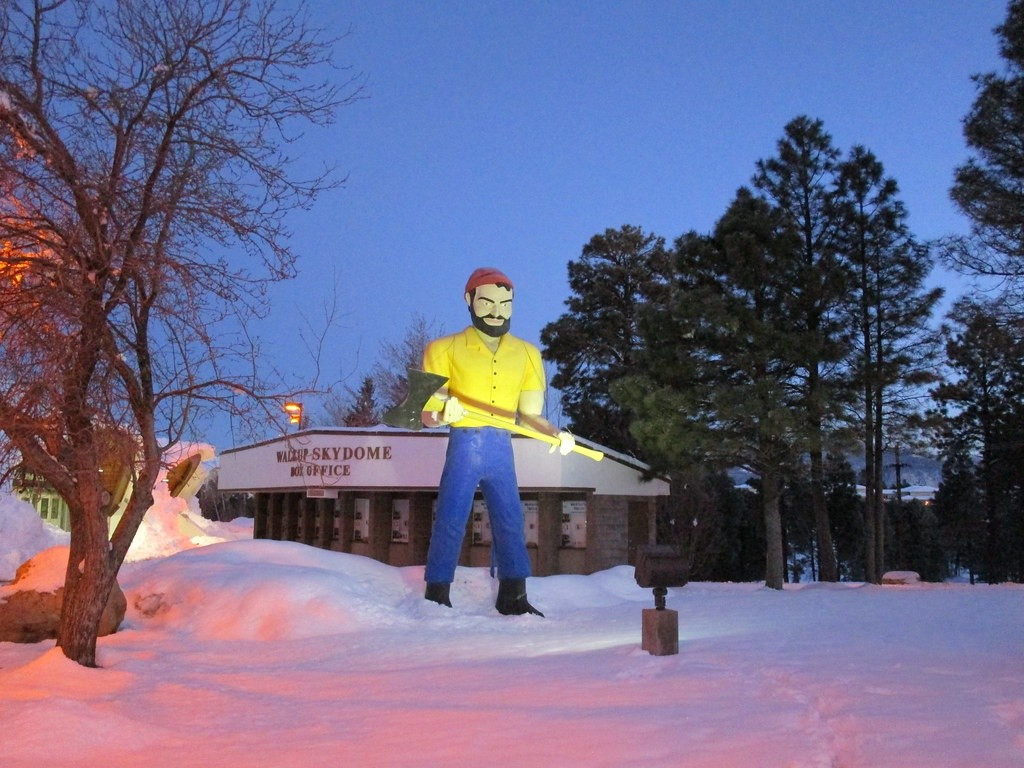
[417,267,576,621]
